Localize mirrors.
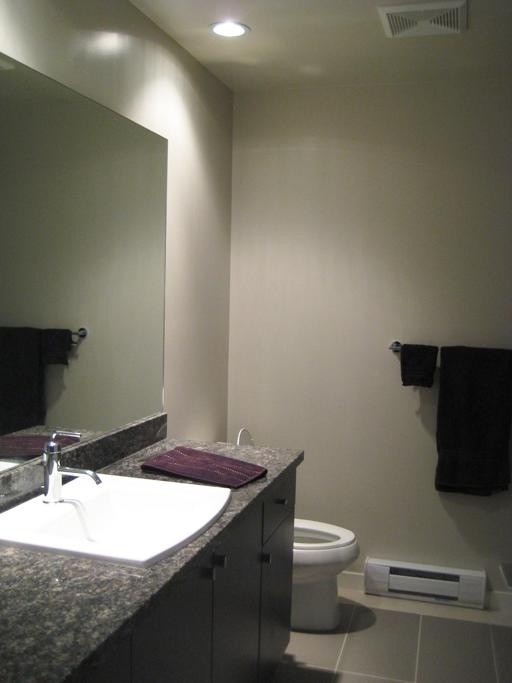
[1,50,170,487]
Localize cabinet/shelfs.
[261,464,298,664]
[127,496,266,681]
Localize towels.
[139,440,271,492]
[3,431,81,462]
[397,341,512,497]
[0,324,75,432]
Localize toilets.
[235,427,360,633]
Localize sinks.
[0,470,231,569]
[41,430,103,503]
[0,460,19,472]
